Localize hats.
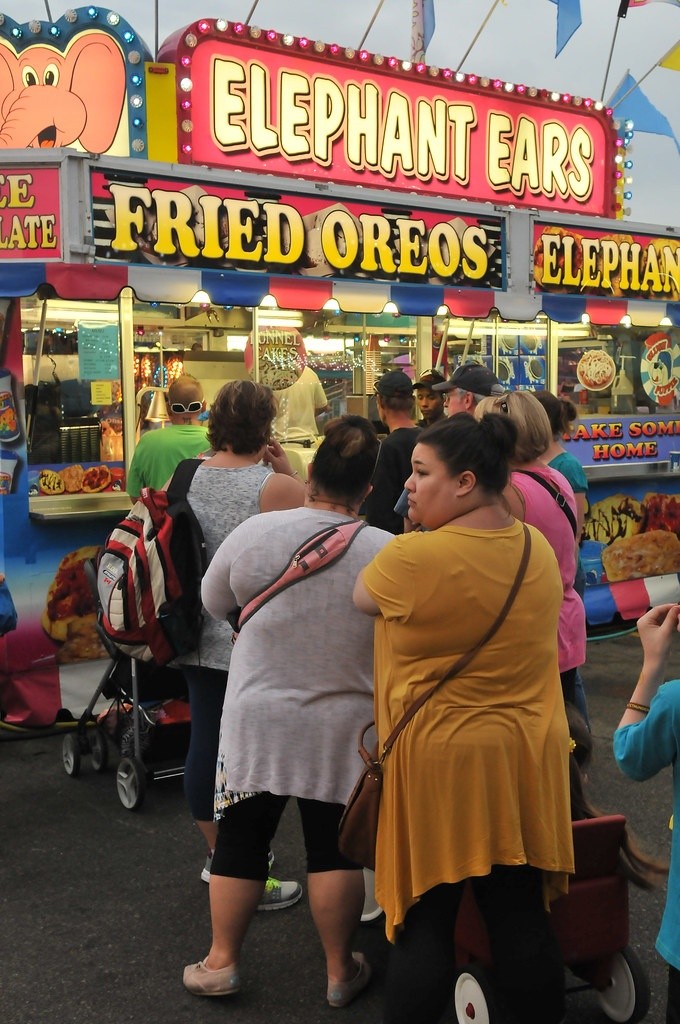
[412,368,447,393]
[370,371,413,402]
[431,363,500,397]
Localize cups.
[110,467,124,492]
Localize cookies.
[498,336,543,381]
[582,492,680,543]
[41,546,110,642]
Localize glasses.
[169,397,205,414]
[494,393,509,413]
[369,438,382,483]
[373,379,381,394]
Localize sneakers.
[183,956,240,994]
[200,848,275,883]
[327,951,369,1007]
[257,876,303,910]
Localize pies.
[38,464,112,493]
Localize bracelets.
[626,702,650,712]
[288,471,298,476]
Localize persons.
[182,416,368,1008]
[275,366,328,446]
[370,360,503,535]
[126,377,212,507]
[159,380,304,913]
[613,602,680,1024]
[353,415,575,1024]
[192,344,202,351]
[473,392,600,821]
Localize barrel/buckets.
[579,539,607,585]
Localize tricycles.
[452,814,650,1023]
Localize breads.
[601,531,680,583]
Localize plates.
[576,350,616,391]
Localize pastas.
[580,350,613,385]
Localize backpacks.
[96,458,208,666]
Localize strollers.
[60,546,189,812]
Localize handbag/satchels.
[336,722,383,872]
